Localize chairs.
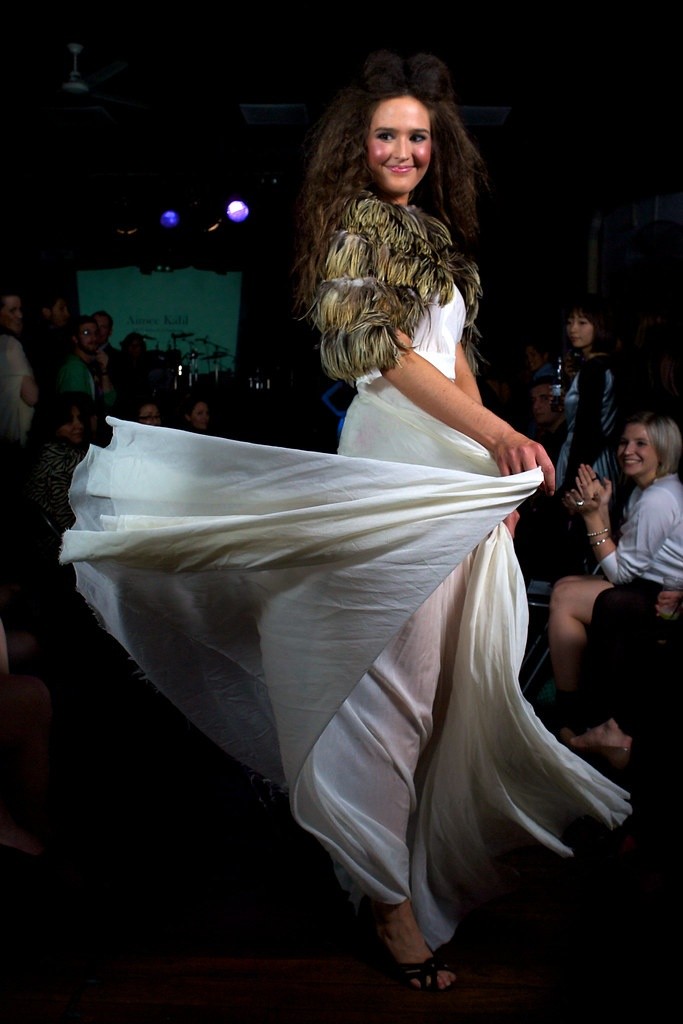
[37,508,67,542]
[519,558,603,695]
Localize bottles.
[550,356,565,412]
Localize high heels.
[373,930,457,991]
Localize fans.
[47,43,153,111]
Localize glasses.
[140,415,160,421]
[79,329,100,337]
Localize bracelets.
[587,527,609,537]
[589,535,610,549]
[102,371,109,376]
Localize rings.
[592,477,598,481]
[577,500,585,506]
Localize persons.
[0,48,683,991]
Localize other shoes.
[560,719,633,771]
